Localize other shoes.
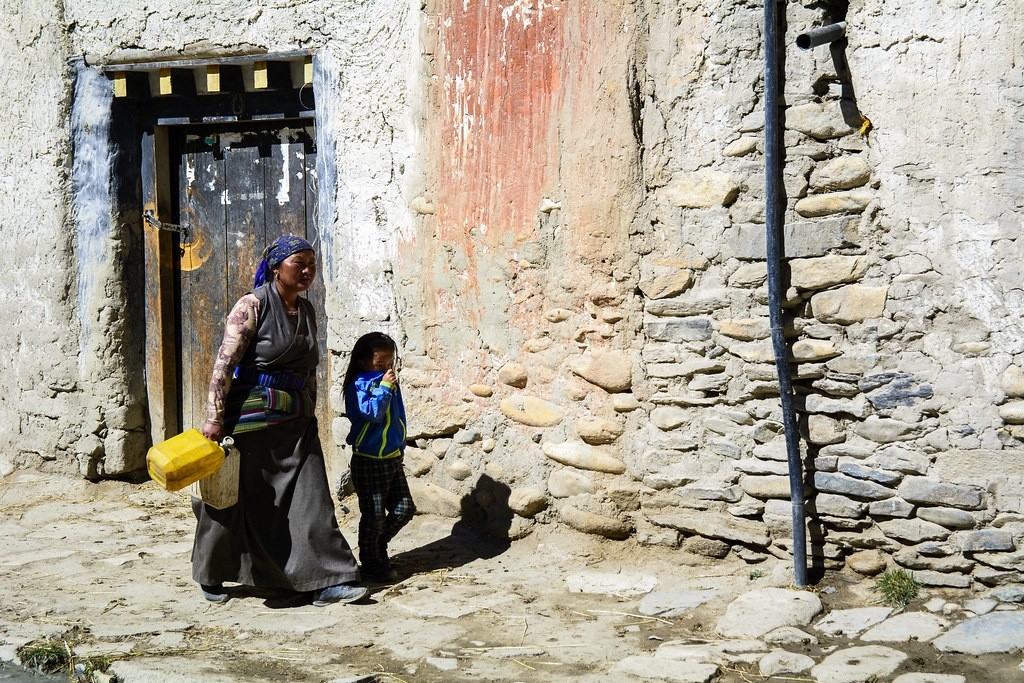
[201,581,229,602]
[361,565,398,583]
[312,585,368,606]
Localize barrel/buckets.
[191,436,240,509]
[146,428,229,491]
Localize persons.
[342,332,417,579]
[190,236,368,607]
